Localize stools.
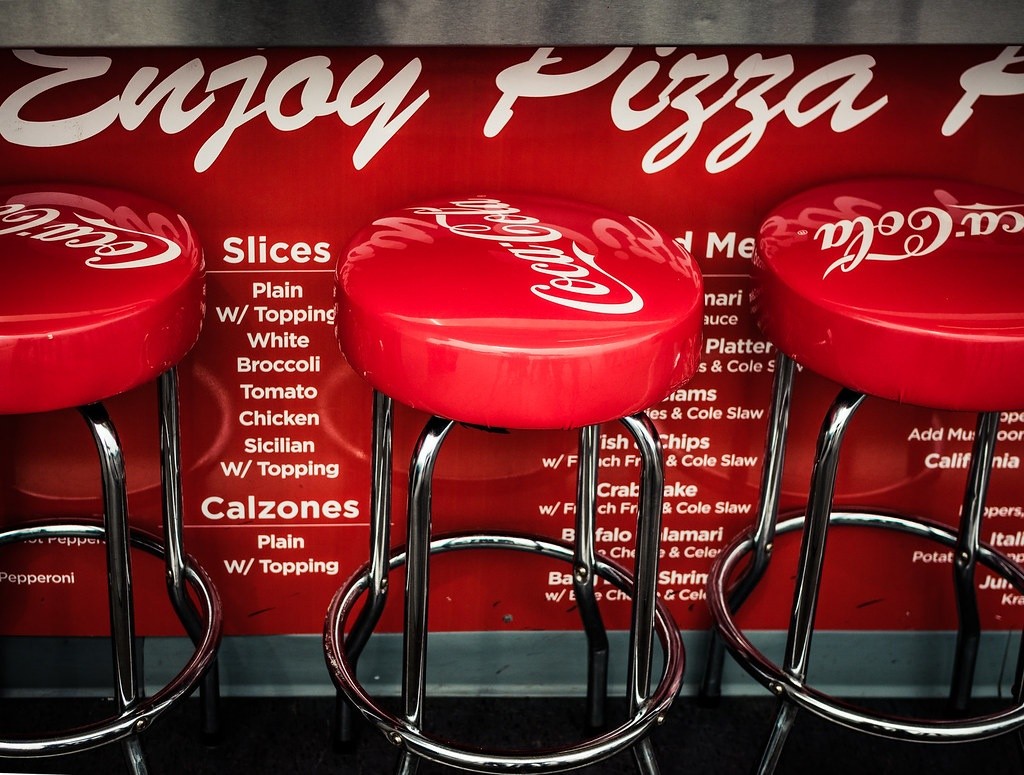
[682,175,1024,775]
[320,192,705,775]
[1,171,221,775]
